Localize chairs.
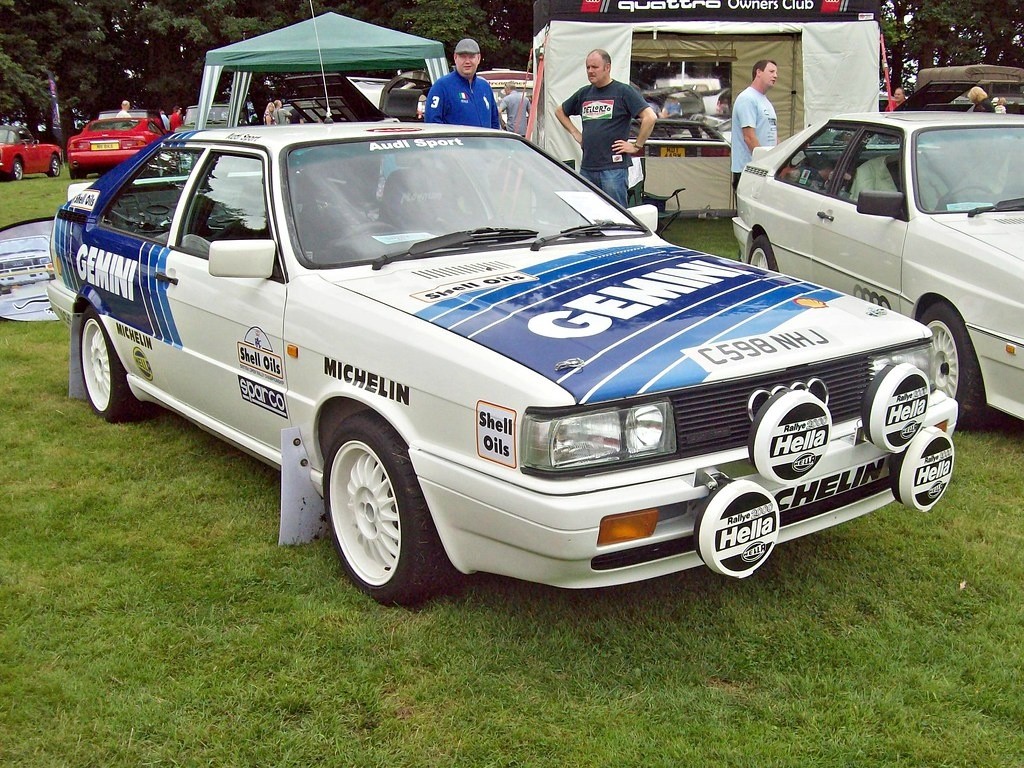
[374,168,448,235]
[628,158,687,242]
[848,153,949,220]
[293,174,359,261]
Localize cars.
[46,121,958,608]
[732,111,1024,429]
[0,126,63,181]
[67,118,164,179]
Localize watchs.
[634,143,643,149]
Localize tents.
[193,12,450,131]
[475,69,534,131]
[514,0,895,218]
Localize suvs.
[174,104,230,133]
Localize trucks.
[917,65,1024,115]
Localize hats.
[455,38,480,55]
[173,105,183,109]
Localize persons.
[288,102,304,124]
[263,102,277,125]
[160,110,168,130]
[555,49,658,210]
[995,97,1007,114]
[885,87,907,112]
[424,39,500,130]
[170,106,182,132]
[662,97,682,119]
[648,100,663,119]
[273,100,292,126]
[967,86,996,113]
[498,82,531,138]
[730,60,787,215]
[116,100,132,118]
